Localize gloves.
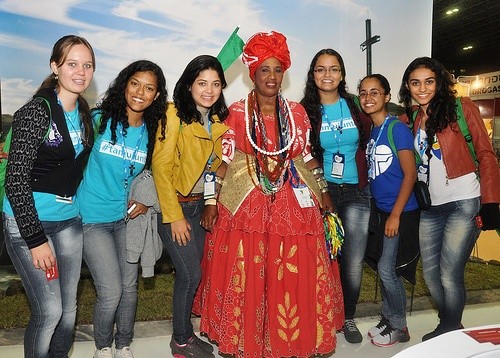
[478,203,500,231]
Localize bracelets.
[315,176,330,195]
[204,191,219,205]
[309,166,324,176]
[214,174,223,187]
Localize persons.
[151,55,231,358]
[190,31,346,358]
[1,34,95,358]
[299,48,421,344]
[356,73,420,348]
[397,57,500,342]
[0,59,168,358]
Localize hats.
[241,31,292,82]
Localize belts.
[176,192,204,202]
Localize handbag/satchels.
[0,97,53,212]
[387,118,423,167]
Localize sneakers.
[367,312,410,347]
[169,330,216,358]
[343,318,362,343]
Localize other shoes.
[115,346,134,358]
[93,347,113,358]
[422,323,464,341]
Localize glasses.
[312,65,341,75]
[358,89,386,97]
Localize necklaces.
[245,89,296,202]
